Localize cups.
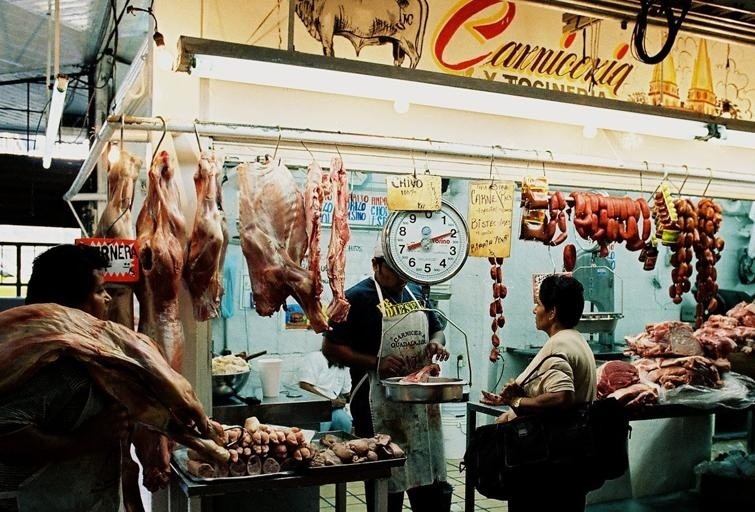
[257,358,281,398]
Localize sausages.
[488,192,725,364]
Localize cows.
[295,0,429,73]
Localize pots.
[211,351,267,401]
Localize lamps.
[36,5,69,173]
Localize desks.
[153,385,408,512]
[465,393,755,512]
[500,344,631,367]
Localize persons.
[1,242,133,511]
[320,243,457,511]
[493,273,600,510]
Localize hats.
[373,235,384,258]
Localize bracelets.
[513,396,523,410]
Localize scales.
[377,196,472,403]
[561,188,624,332]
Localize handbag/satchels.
[461,357,633,502]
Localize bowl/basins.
[380,377,467,400]
[572,313,620,334]
